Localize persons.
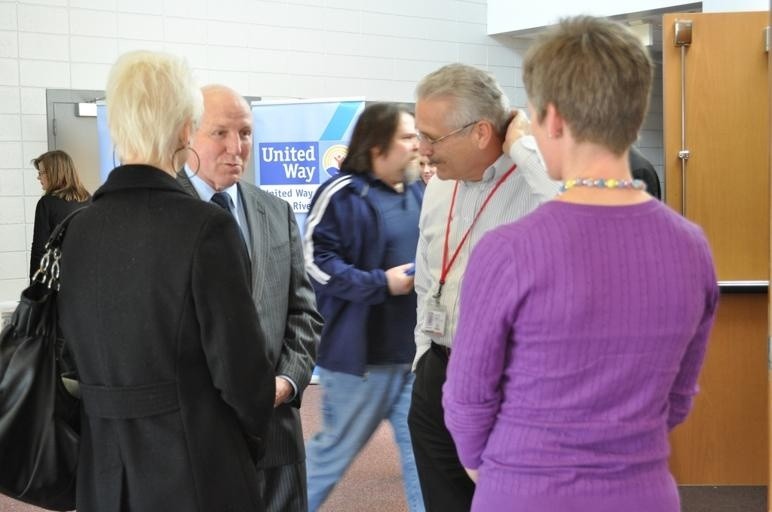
[406,61,567,511]
[627,144,662,202]
[417,151,437,184]
[172,81,326,511]
[438,10,719,511]
[44,44,276,511]
[26,151,94,289]
[298,100,426,511]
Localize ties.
[209,190,255,299]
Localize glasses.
[415,119,496,147]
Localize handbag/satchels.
[0,202,95,512]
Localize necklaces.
[553,176,651,190]
